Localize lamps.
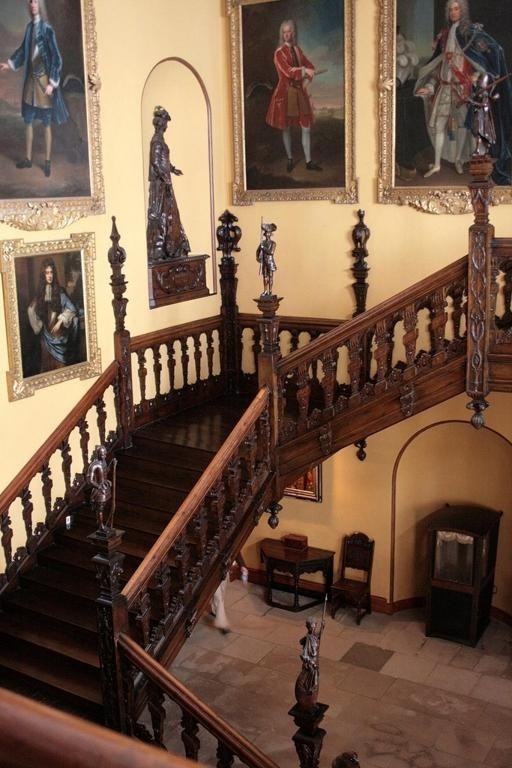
[265,501,282,529]
[354,438,366,460]
[465,396,489,431]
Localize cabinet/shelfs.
[425,503,503,649]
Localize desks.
[260,538,335,612]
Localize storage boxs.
[284,534,308,553]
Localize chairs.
[327,531,374,625]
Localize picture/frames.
[0,0,107,231]
[376,0,512,215]
[283,464,322,504]
[222,0,357,205]
[0,232,103,402]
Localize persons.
[0,1,71,181]
[412,1,511,187]
[255,222,278,294]
[86,442,119,532]
[207,547,249,635]
[144,104,193,266]
[24,255,84,373]
[263,18,323,176]
[293,616,324,705]
